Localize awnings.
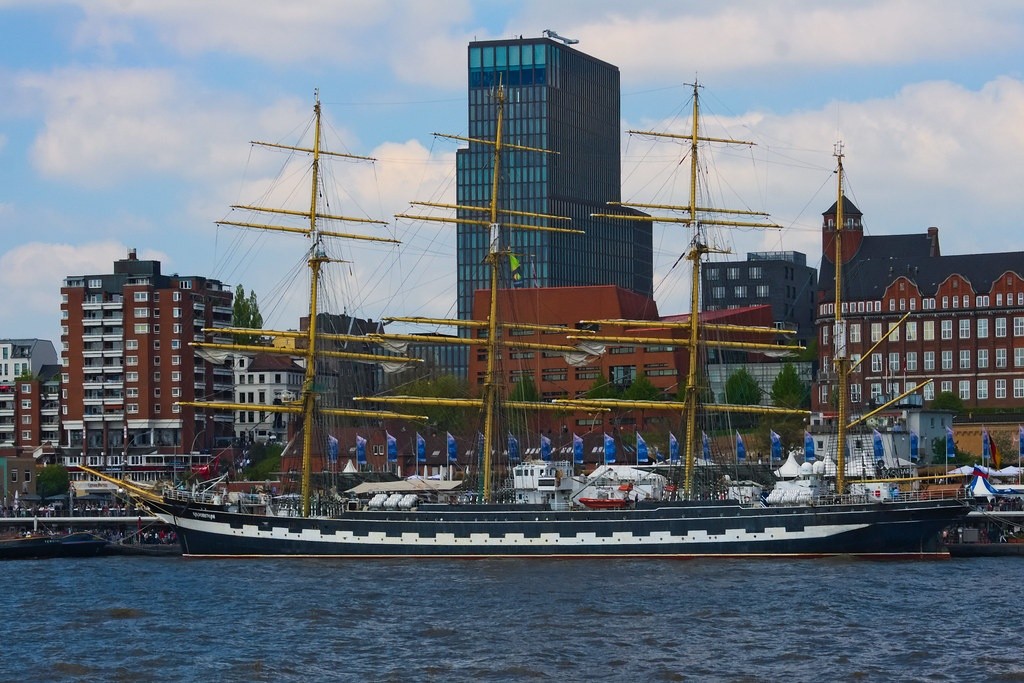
[948,464,1024,496]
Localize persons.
[2,500,177,545]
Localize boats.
[0,487,111,559]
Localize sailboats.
[79,75,978,561]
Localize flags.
[509,256,521,283]
[531,257,539,289]
[325,426,1024,461]
